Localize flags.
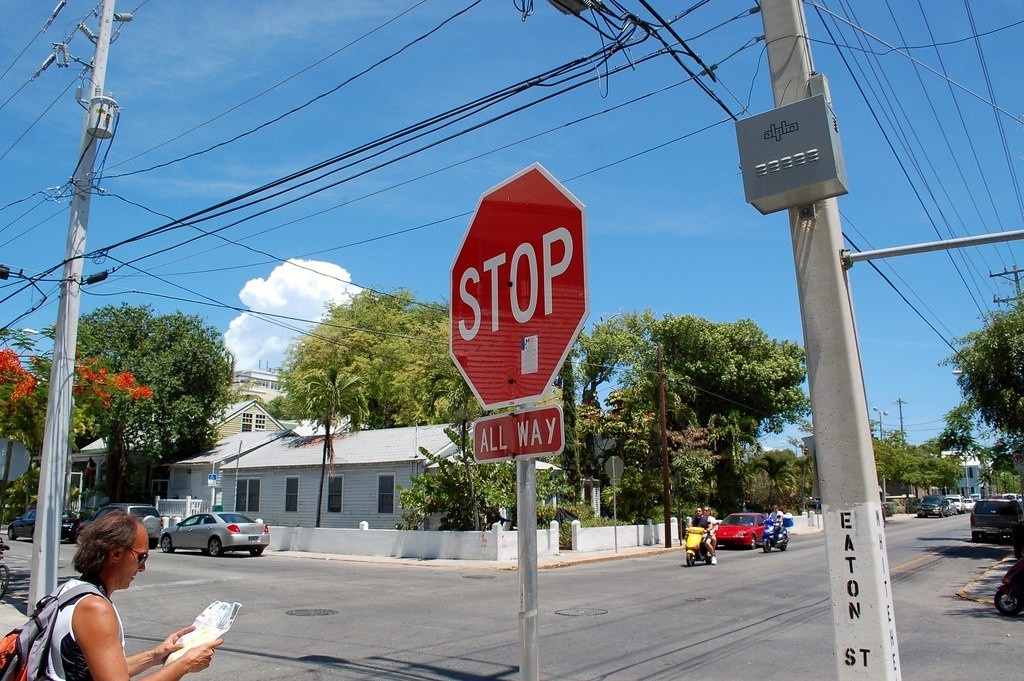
[85,457,96,477]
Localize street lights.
[872,407,891,503]
[956,451,979,498]
[780,439,798,459]
[801,447,809,508]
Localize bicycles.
[0,536,10,601]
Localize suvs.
[915,495,950,519]
[73,502,164,549]
[969,496,1024,542]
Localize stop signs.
[447,163,591,411]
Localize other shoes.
[711,557,717,564]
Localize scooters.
[760,514,794,553]
[992,546,1024,617]
[682,519,718,567]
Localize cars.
[714,512,768,550]
[7,507,83,543]
[805,496,821,510]
[945,493,1024,514]
[157,512,271,558]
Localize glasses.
[126,547,148,564]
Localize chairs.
[204,518,213,524]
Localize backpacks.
[0,582,111,681]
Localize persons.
[685,507,718,564]
[768,505,786,533]
[37,510,224,681]
